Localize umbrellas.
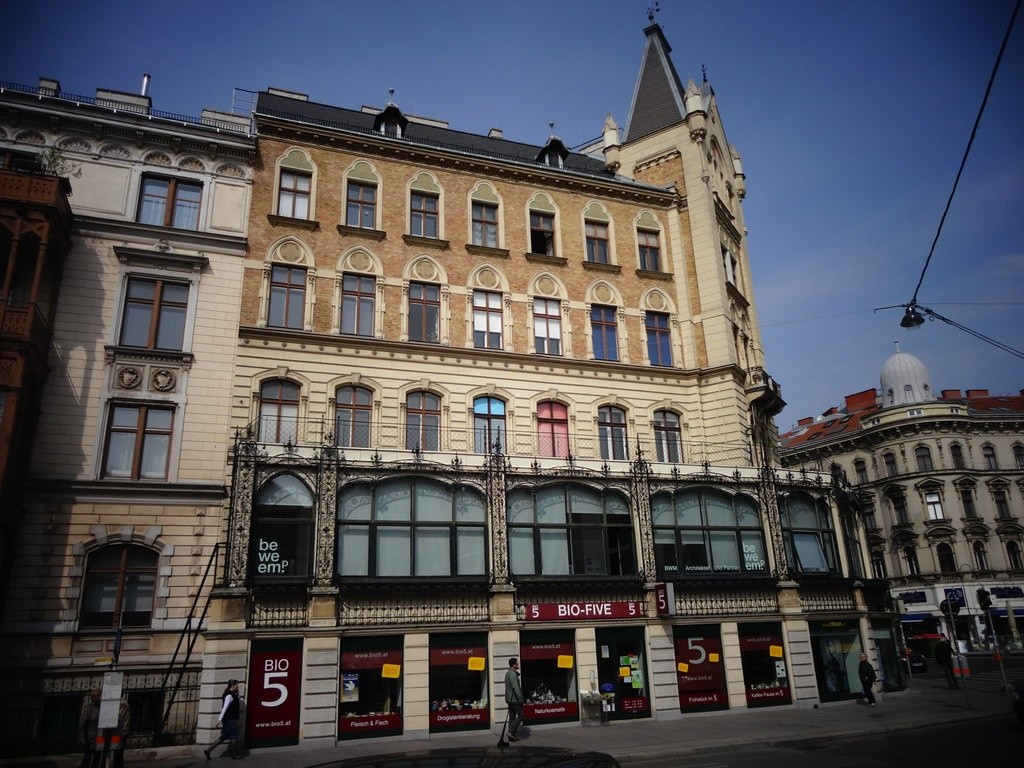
[1005,600,1020,642]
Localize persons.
[858,652,877,707]
[936,633,961,690]
[79,688,131,768]
[204,679,242,760]
[505,658,526,742]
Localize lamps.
[590,669,595,684]
[900,307,925,331]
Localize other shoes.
[506,732,521,742]
[231,754,245,760]
[203,748,213,760]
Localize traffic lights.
[978,588,993,609]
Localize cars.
[901,651,929,672]
[986,635,1001,651]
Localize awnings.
[898,613,931,622]
[990,608,1024,617]
